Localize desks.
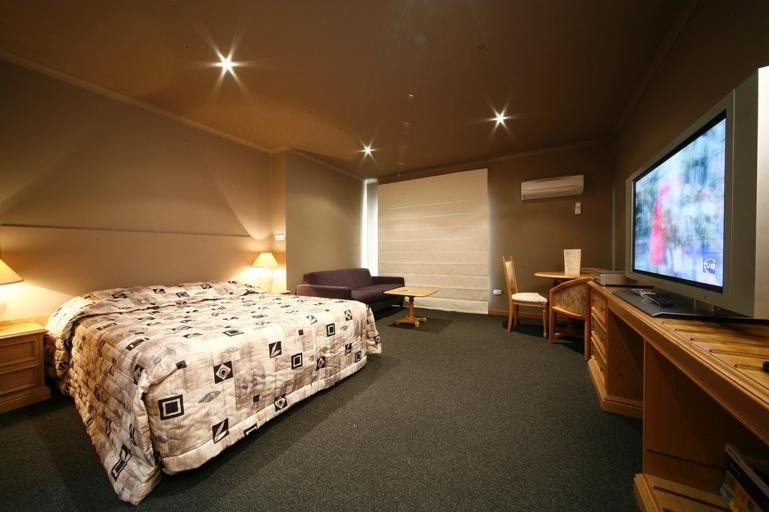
[533,271,581,287]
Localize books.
[718,444,769,512]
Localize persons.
[649,185,671,274]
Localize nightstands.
[280,290,291,295]
[0,321,51,412]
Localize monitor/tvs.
[611,65,769,321]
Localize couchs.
[296,268,404,312]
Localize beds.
[43,280,383,506]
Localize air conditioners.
[520,174,585,200]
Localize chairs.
[503,255,547,338]
[548,275,599,360]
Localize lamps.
[252,252,279,291]
[0,259,24,324]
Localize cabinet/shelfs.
[586,280,769,512]
